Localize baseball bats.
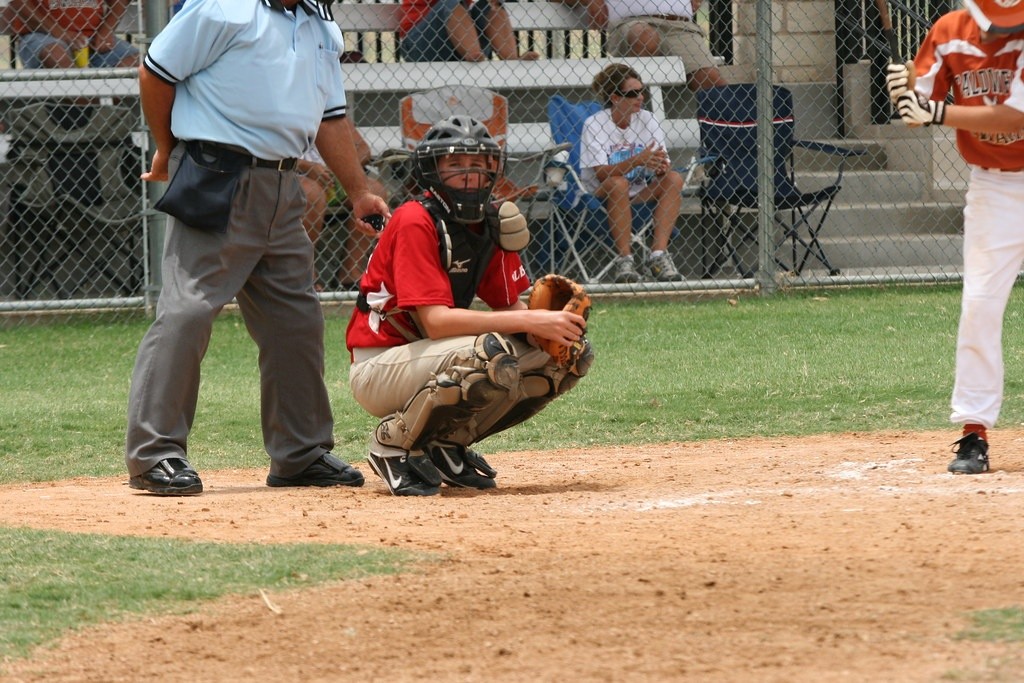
[876,0,923,128]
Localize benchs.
[0,0,760,220]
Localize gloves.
[899,91,946,125]
[887,60,917,105]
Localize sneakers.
[947,432,990,474]
[425,441,498,489]
[646,249,682,281]
[266,453,365,487]
[367,452,442,497]
[129,458,203,494]
[615,253,638,283]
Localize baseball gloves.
[526,274,594,372]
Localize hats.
[963,0,1024,35]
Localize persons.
[0,0,140,69]
[124,0,392,497]
[579,63,684,283]
[885,0,1024,475]
[345,115,594,497]
[398,0,540,62]
[294,111,390,292]
[550,0,728,92]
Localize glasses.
[617,88,644,98]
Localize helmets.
[414,114,507,221]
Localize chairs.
[696,84,867,279]
[6,102,151,301]
[9,29,132,69]
[368,84,719,284]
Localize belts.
[629,14,689,22]
[982,167,1022,172]
[199,142,295,171]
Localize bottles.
[74,38,90,68]
[610,142,644,178]
